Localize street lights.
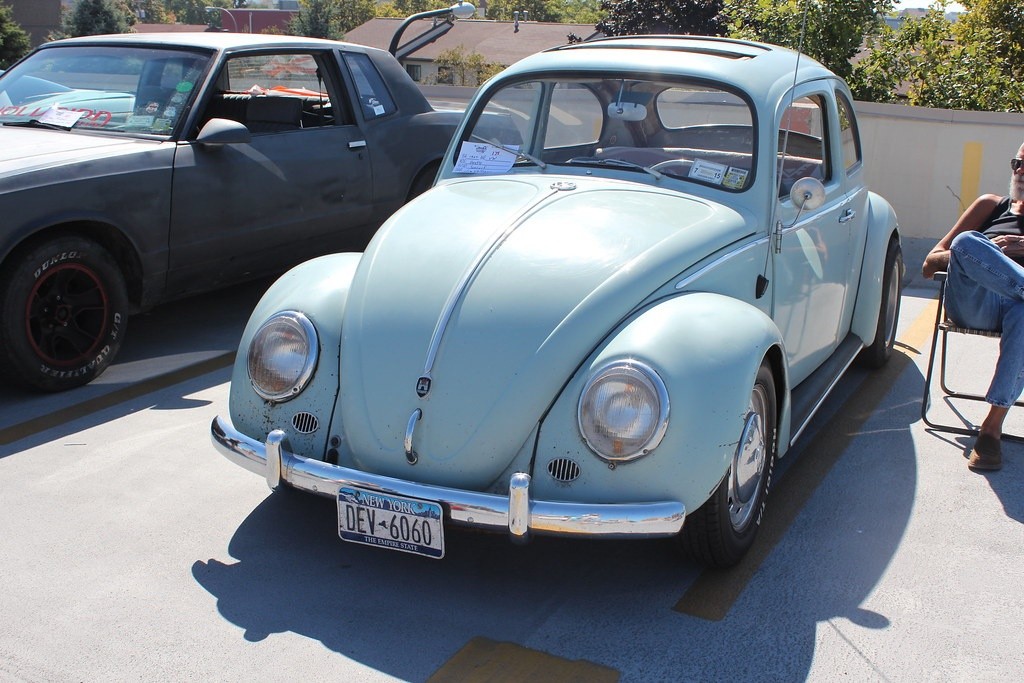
[204,6,239,30]
[389,2,476,56]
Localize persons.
[922,141,1024,470]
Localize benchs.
[196,91,304,135]
[593,144,824,199]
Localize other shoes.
[968,434,1002,470]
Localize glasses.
[1010,158,1024,170]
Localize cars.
[0,32,525,394]
[211,33,907,569]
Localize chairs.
[922,270,1024,444]
[139,84,168,113]
